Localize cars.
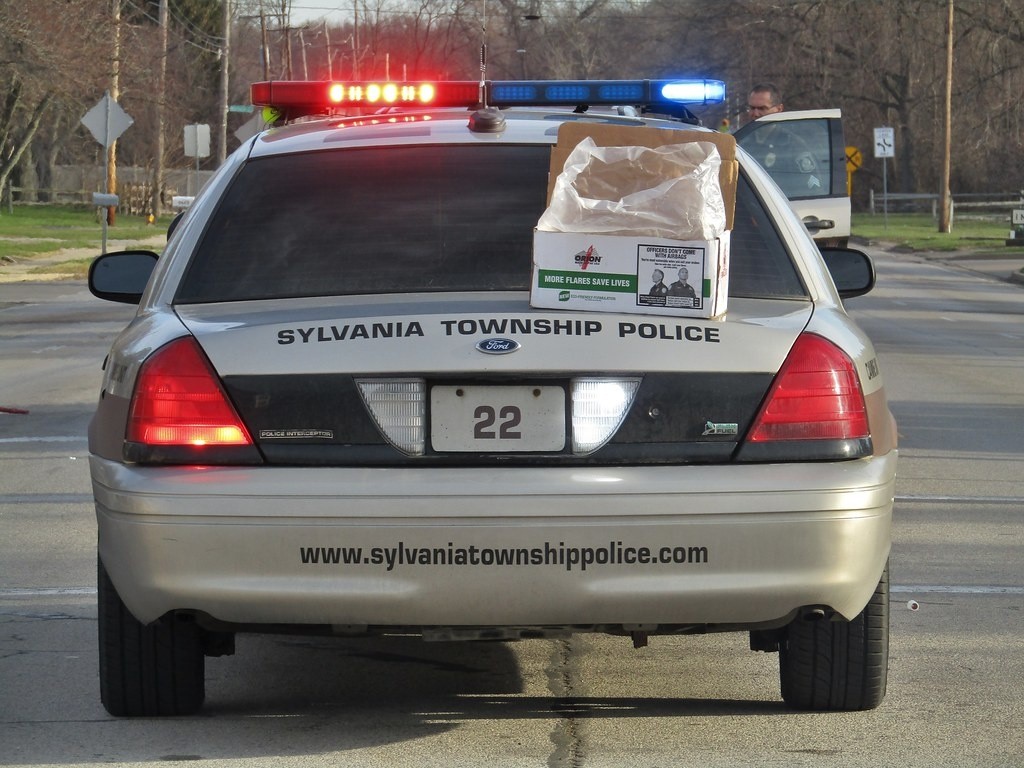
[85,79,895,720]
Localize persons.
[736,83,823,194]
[648,268,697,309]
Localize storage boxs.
[529,120,739,319]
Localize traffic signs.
[873,128,895,157]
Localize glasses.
[745,104,777,113]
